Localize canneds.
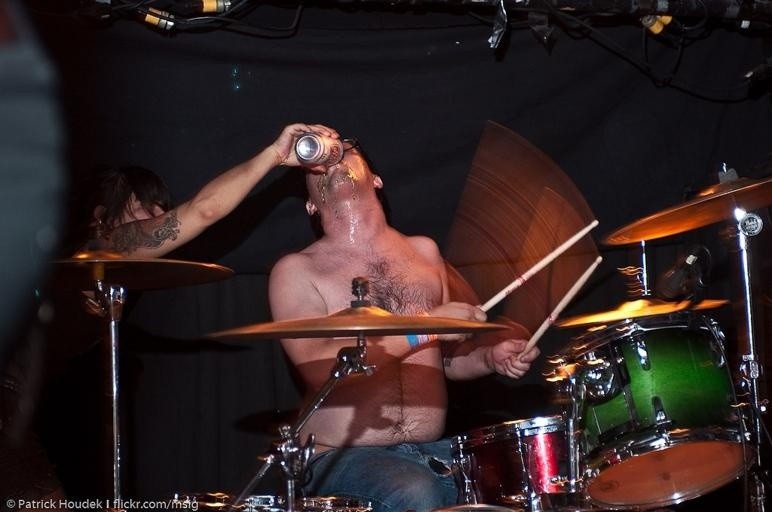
[294,133,344,166]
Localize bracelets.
[418,334,429,347]
[428,334,434,343]
[406,334,420,352]
[433,334,437,341]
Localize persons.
[267,135,542,512]
[34,122,341,512]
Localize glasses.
[341,135,364,155]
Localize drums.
[558,313,758,511]
[450,410,579,509]
[177,492,378,510]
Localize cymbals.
[551,298,734,329]
[602,175,771,246]
[200,304,510,344]
[36,249,233,293]
